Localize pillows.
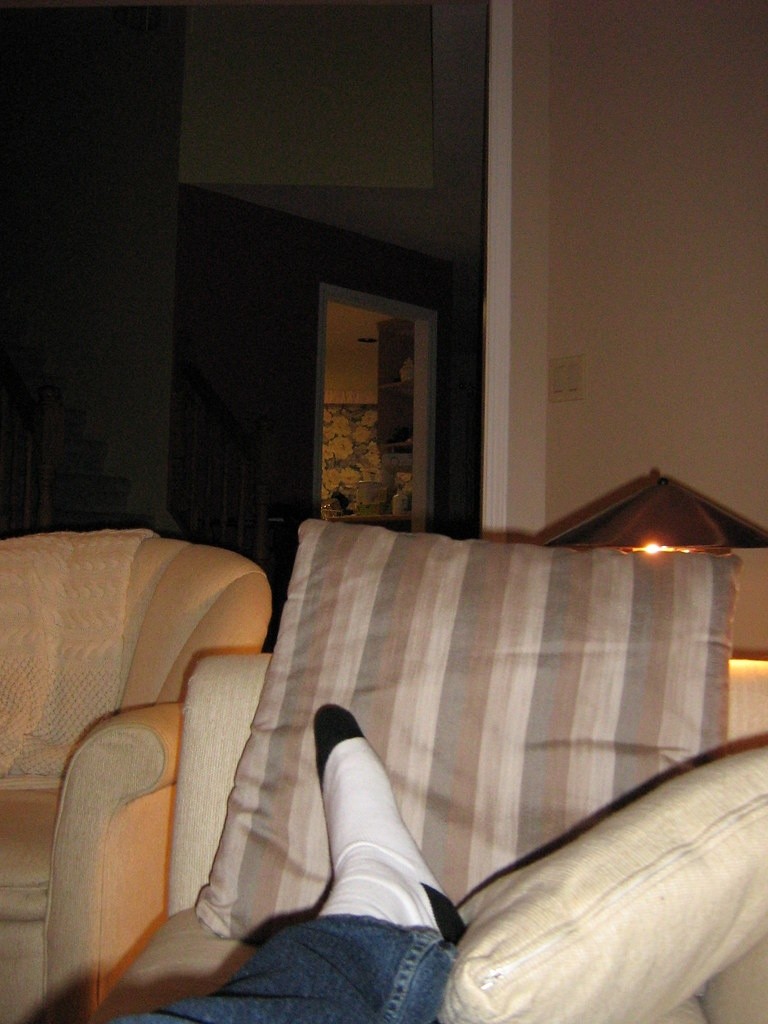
[210,519,768,1024]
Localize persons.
[104,704,466,1024]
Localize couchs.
[0,524,274,1024]
[91,652,768,1024]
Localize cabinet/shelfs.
[377,318,414,450]
